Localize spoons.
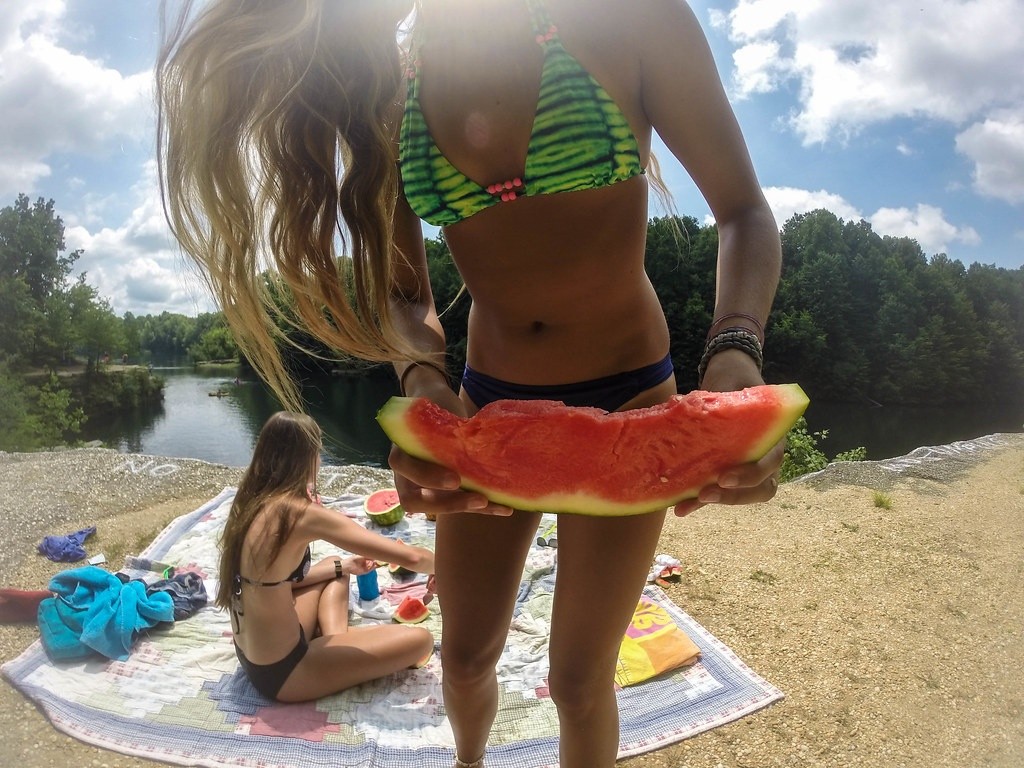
[423,590,434,605]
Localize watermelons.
[364,488,405,526]
[389,538,415,574]
[376,382,809,517]
[392,596,431,624]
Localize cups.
[356,569,379,601]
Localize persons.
[215,410,439,704]
[154,0,789,768]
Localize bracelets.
[698,312,765,388]
[333,560,342,578]
[400,360,453,398]
